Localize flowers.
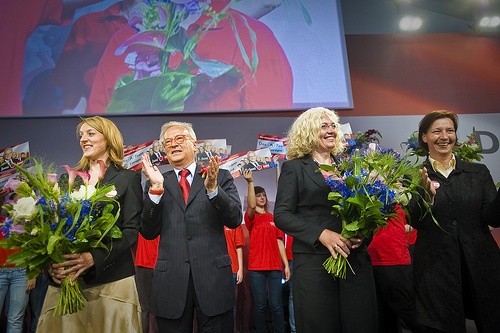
[105,0,314,115]
[0,151,123,319]
[320,126,486,280]
[199,165,211,177]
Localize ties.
[178,169,191,206]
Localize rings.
[333,245,337,249]
[50,273,54,277]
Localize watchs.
[149,187,164,195]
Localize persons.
[274,107,380,333]
[223,225,246,288]
[140,122,243,333]
[241,168,294,333]
[369,202,419,333]
[146,139,169,164]
[0,148,29,171]
[36,116,143,333]
[133,231,160,311]
[238,151,277,172]
[410,110,500,333]
[0,191,51,333]
[195,141,225,165]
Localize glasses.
[320,123,338,130]
[163,136,195,148]
